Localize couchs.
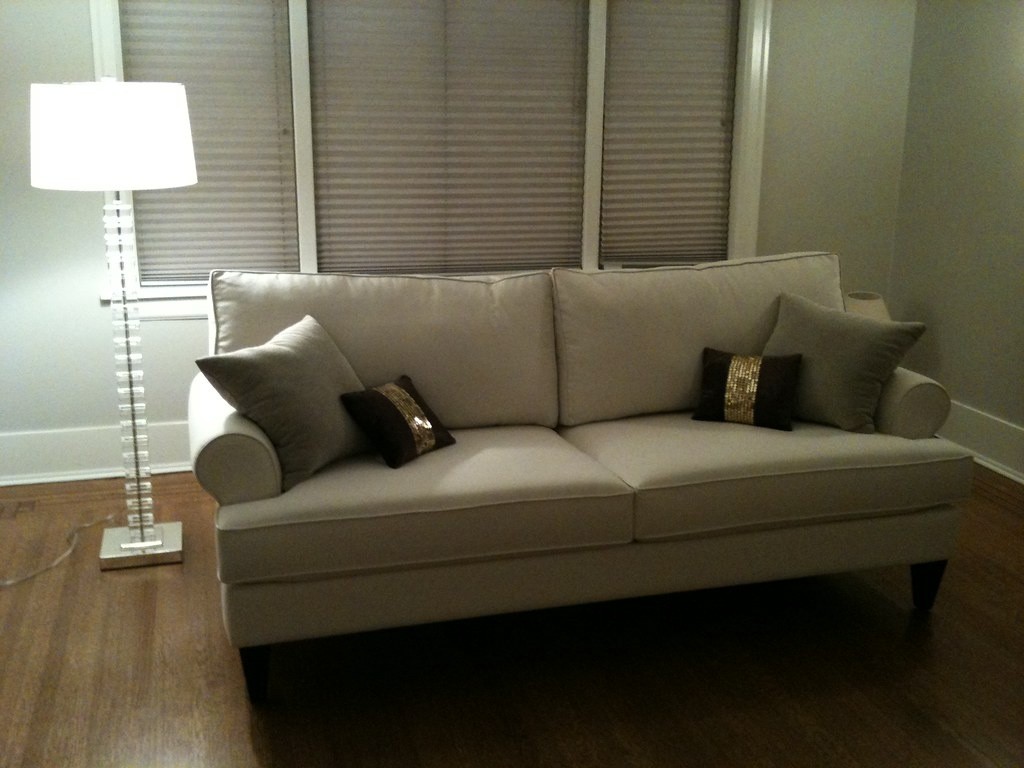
[189,249,974,708]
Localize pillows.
[692,347,803,431]
[762,294,926,433]
[340,375,456,470]
[196,314,367,492]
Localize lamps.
[29,77,197,570]
